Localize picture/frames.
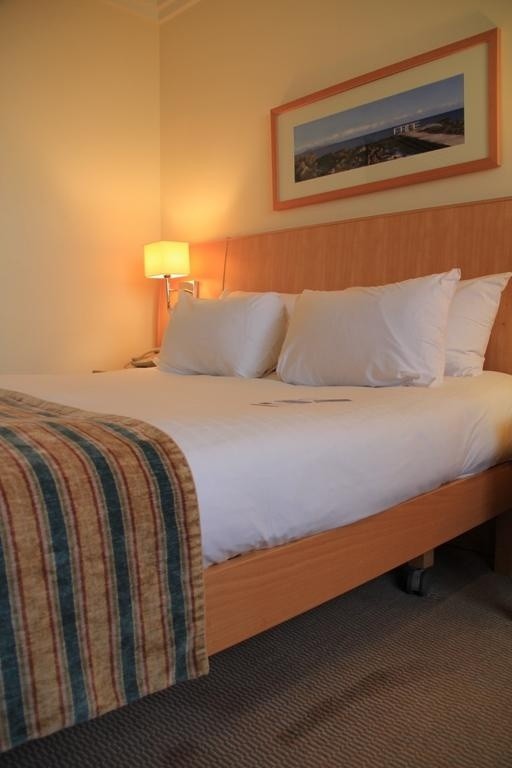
[271,26,502,210]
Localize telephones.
[130,348,160,367]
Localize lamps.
[143,240,193,313]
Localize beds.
[0,196,512,756]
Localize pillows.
[218,288,301,327]
[275,266,462,388]
[444,271,512,380]
[151,287,288,378]
